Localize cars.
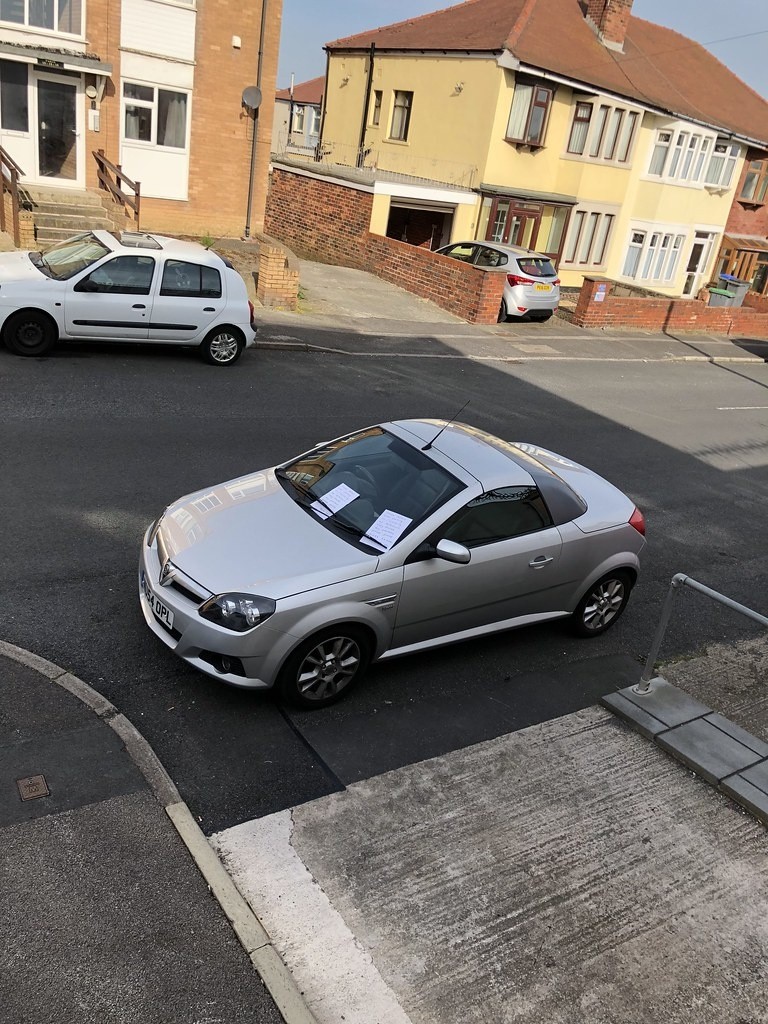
[0,232,259,364]
[138,416,647,711]
[434,242,562,323]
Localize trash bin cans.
[716,274,736,289]
[726,279,752,307]
[709,286,735,309]
